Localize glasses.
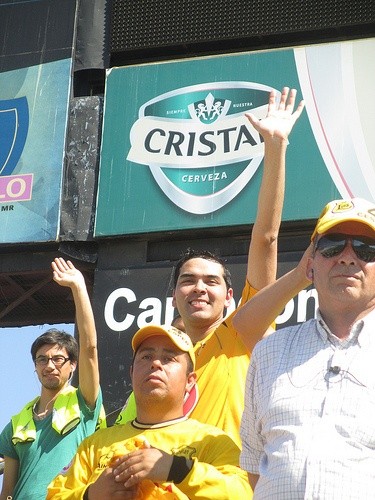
[314,233,375,263]
[35,356,73,365]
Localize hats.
[311,199,375,245]
[131,324,195,372]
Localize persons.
[171,87,307,452]
[237,198,375,500]
[171,313,187,333]
[0,256,102,500]
[44,323,253,500]
[231,241,315,356]
